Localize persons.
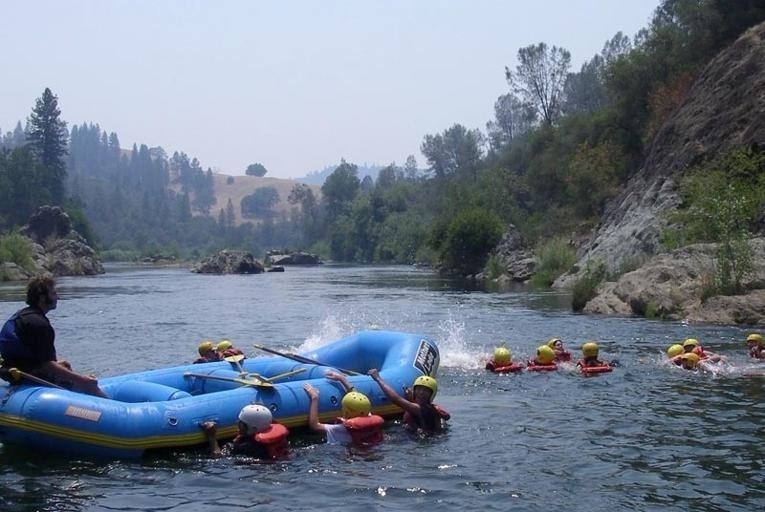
[487,344,520,374]
[546,337,570,362]
[668,335,722,375]
[576,339,614,373]
[746,330,765,360]
[0,270,116,401]
[302,369,385,447]
[199,403,292,460]
[190,339,245,365]
[367,366,449,430]
[527,345,559,374]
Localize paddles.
[225,354,247,372]
[183,373,274,388]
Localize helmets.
[412,376,438,403]
[342,392,372,418]
[239,405,273,435]
[217,341,233,352]
[747,334,762,344]
[199,343,213,355]
[668,339,701,367]
[495,349,511,366]
[536,339,562,362]
[582,343,600,359]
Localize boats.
[0,332,440,464]
